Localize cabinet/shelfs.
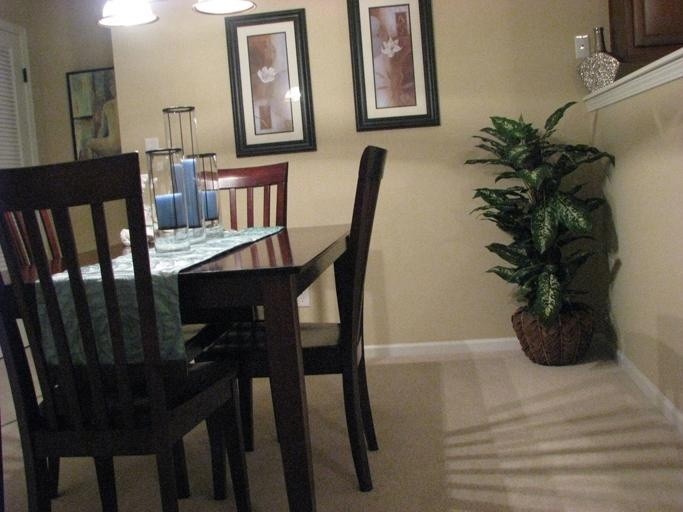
[607,0,683,82]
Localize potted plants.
[462,97,618,366]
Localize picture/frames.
[346,0,442,132]
[224,8,319,158]
[65,67,121,160]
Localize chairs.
[198,162,288,227]
[184,145,389,501]
[0,153,252,512]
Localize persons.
[79,75,123,161]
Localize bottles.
[183,152,226,237]
[160,103,208,245]
[144,146,194,259]
